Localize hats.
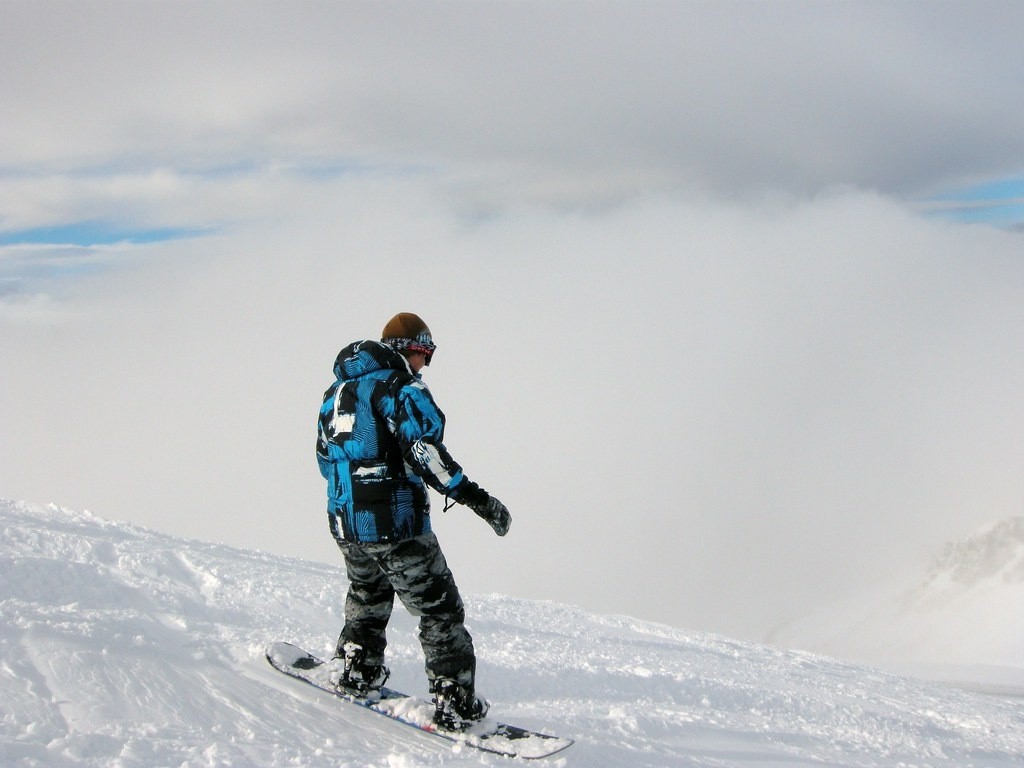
[382,312,432,344]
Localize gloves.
[468,488,512,536]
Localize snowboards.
[263,638,578,764]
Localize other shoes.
[339,658,390,697]
[433,680,490,733]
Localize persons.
[316,312,512,734]
